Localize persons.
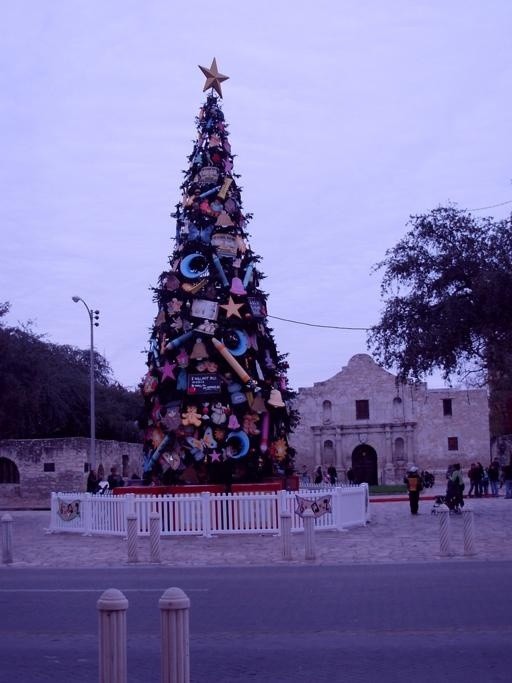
[301,462,338,485]
[132,474,141,479]
[444,457,512,514]
[347,466,355,484]
[107,467,125,490]
[403,466,434,515]
[87,469,102,492]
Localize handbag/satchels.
[448,477,459,489]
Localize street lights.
[73,296,100,476]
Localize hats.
[410,467,419,472]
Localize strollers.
[430,481,461,514]
